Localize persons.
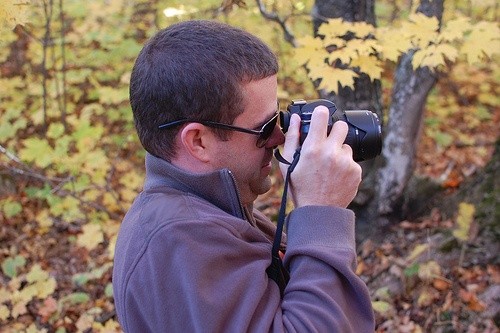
[111,21,375,333]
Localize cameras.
[280,99,383,163]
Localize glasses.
[157,100,280,149]
